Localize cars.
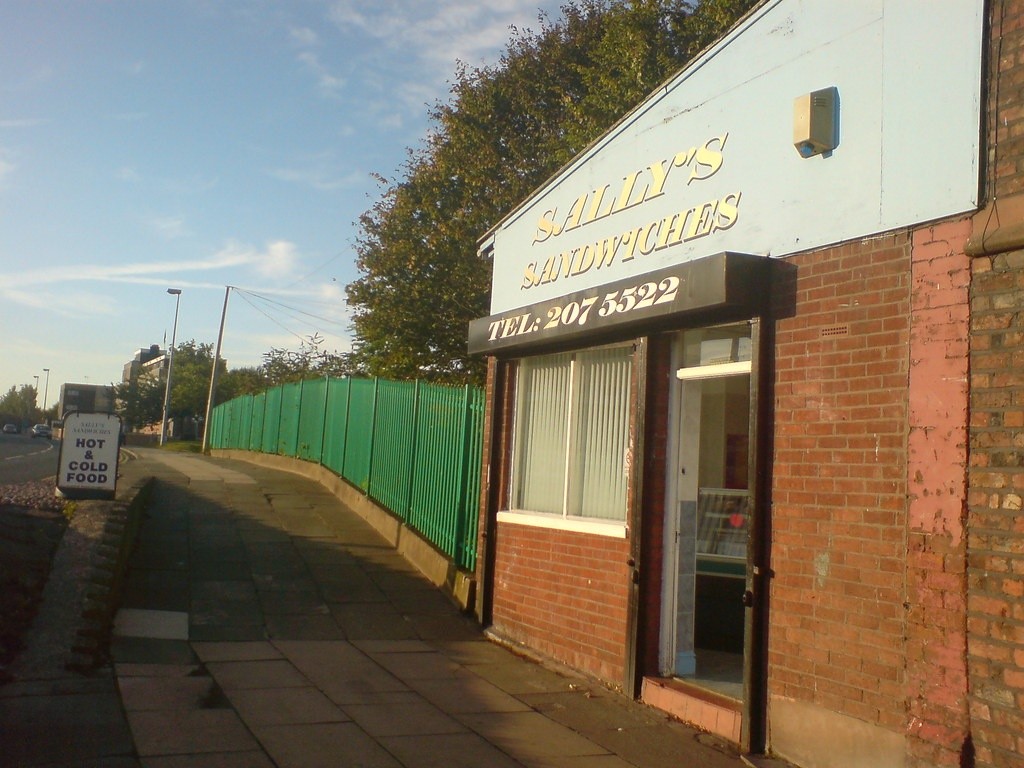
[31,424,53,442]
[3,424,17,434]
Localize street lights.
[33,375,39,390]
[160,288,182,446]
[42,368,50,412]
